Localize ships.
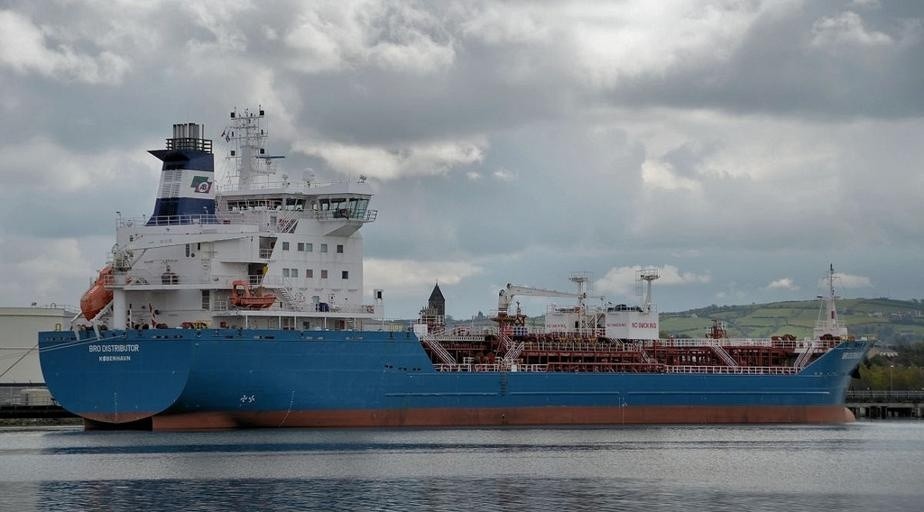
[33,104,868,434]
